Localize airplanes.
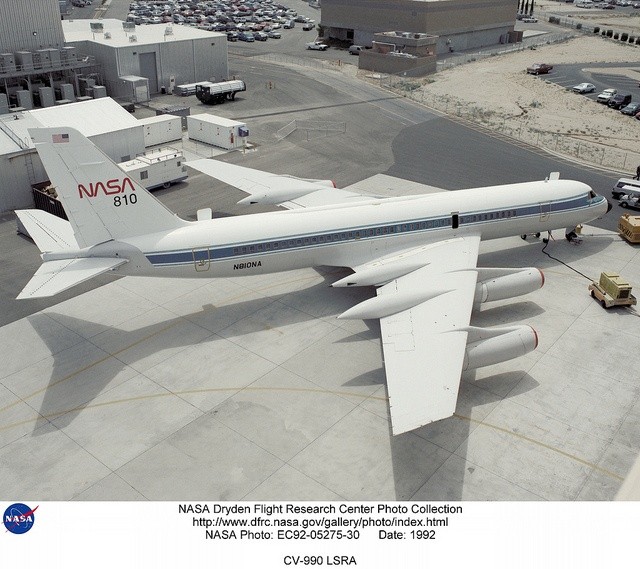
[15,127,612,437]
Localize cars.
[618,194,640,210]
[126,1,320,41]
[620,101,640,116]
[572,83,596,94]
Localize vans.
[612,177,640,199]
[349,46,365,55]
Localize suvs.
[607,92,632,110]
[597,89,617,104]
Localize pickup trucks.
[306,41,329,51]
[527,63,553,75]
